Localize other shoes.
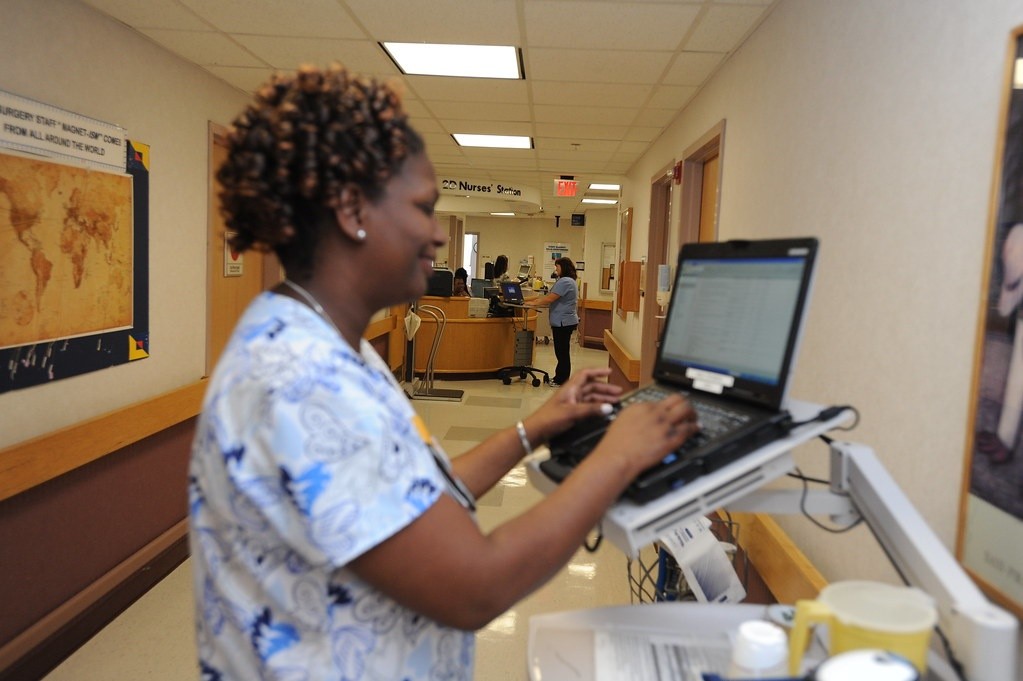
[545,378,562,387]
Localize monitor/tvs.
[483,286,498,298]
[471,278,493,298]
[518,264,531,278]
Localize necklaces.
[283,276,339,333]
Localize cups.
[791,579,937,681]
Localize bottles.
[528,275,543,289]
[724,618,793,680]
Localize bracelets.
[517,420,533,456]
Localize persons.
[188,64,701,681]
[454,276,470,296]
[523,257,579,387]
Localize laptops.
[500,282,524,305]
[539,237,819,506]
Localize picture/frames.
[952,27,1023,624]
